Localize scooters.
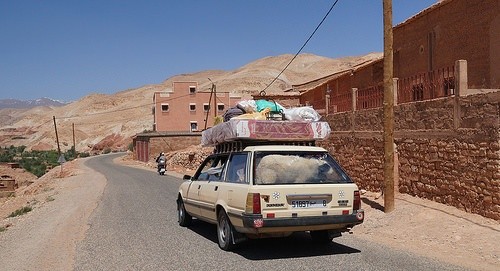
[154,157,166,175]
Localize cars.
[176,139,365,251]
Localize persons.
[156,152,167,172]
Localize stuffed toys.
[254,155,334,185]
